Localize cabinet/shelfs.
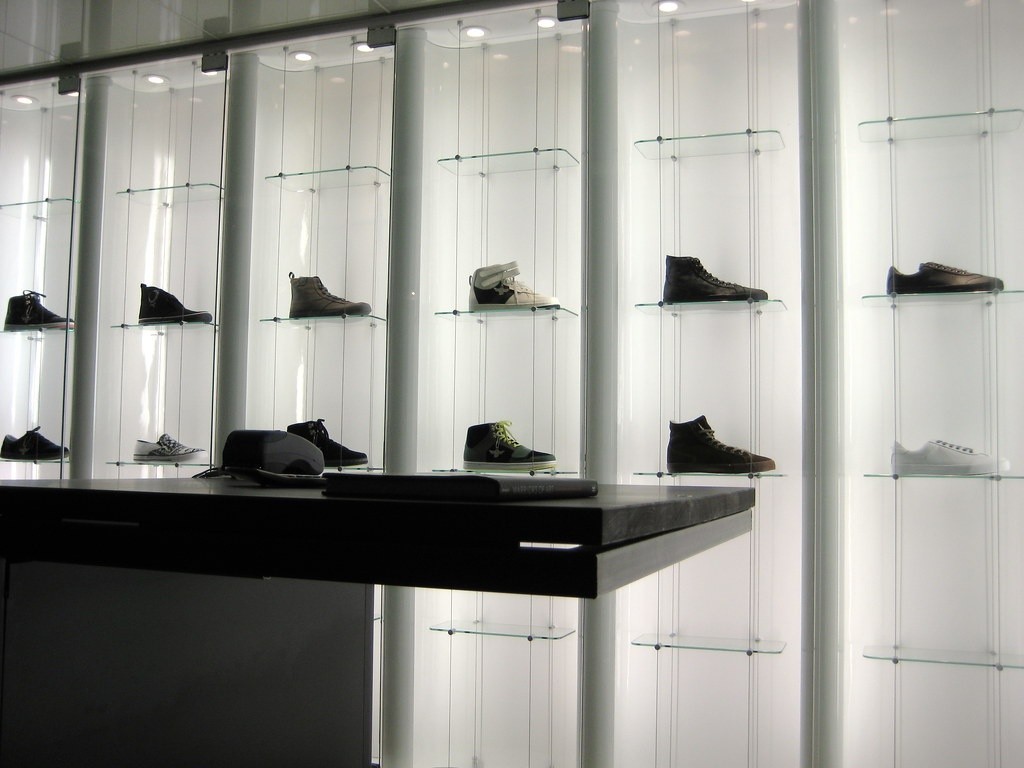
[0,108,1024,670]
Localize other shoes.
[134,434,208,462]
[287,419,368,467]
[138,283,212,322]
[288,272,372,318]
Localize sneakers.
[464,420,557,469]
[887,262,1004,292]
[468,261,561,310]
[663,255,768,302]
[666,415,775,473]
[1,426,69,461]
[892,440,1009,475]
[4,290,74,329]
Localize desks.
[0,475,756,768]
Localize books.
[322,471,598,504]
